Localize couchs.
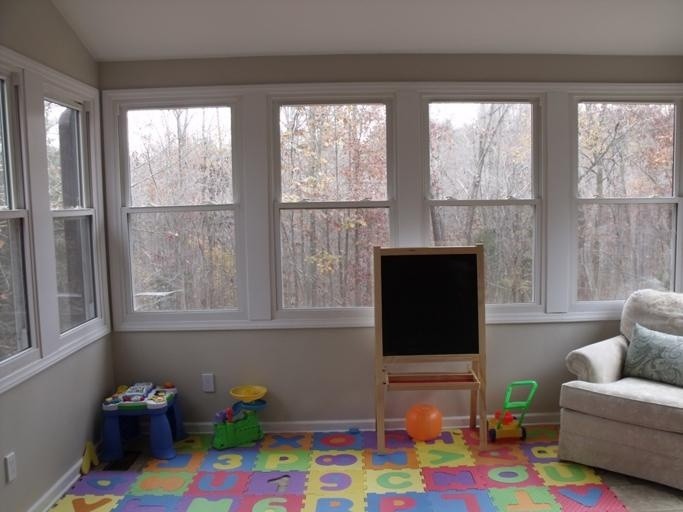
[559,288,683,491]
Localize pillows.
[623,324,683,387]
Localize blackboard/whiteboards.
[374,243,487,365]
